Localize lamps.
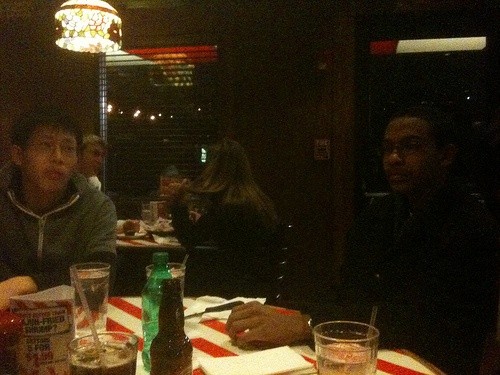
[55,0,123,53]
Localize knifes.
[183,301,244,319]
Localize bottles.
[150,279,194,375]
[141,252,172,371]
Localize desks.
[75,297,444,375]
[116,220,218,254]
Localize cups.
[145,262,186,305]
[66,331,139,375]
[312,320,380,375]
[154,201,168,220]
[141,210,152,226]
[69,262,111,339]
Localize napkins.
[198,346,313,375]
[183,295,266,323]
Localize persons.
[228,106,495,375]
[169,137,278,296]
[0,102,118,311]
[78,133,107,186]
[146,166,198,222]
